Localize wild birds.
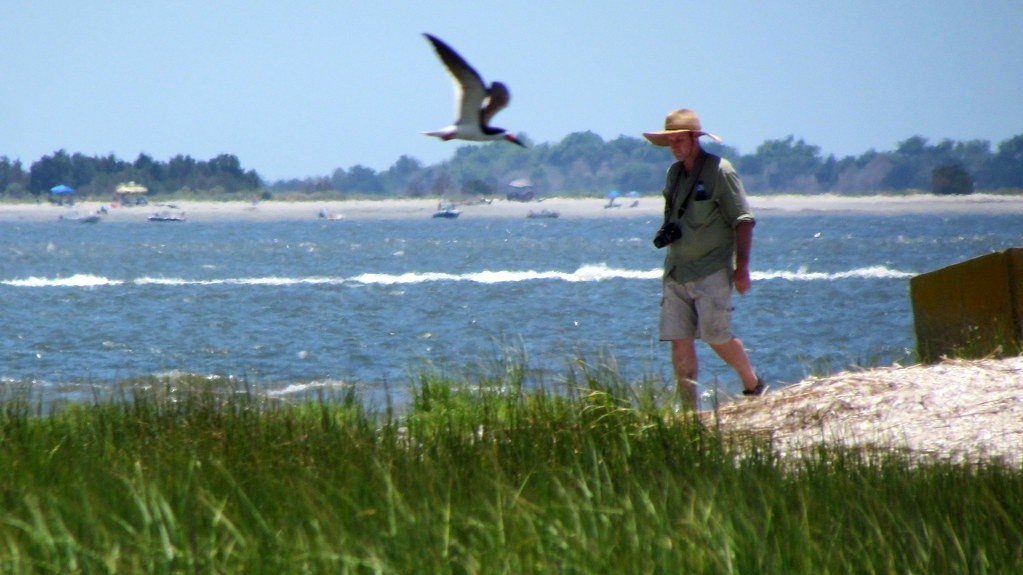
[421,31,528,149]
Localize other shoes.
[743,376,770,397]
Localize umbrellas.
[51,185,75,196]
[117,182,148,193]
[509,179,536,188]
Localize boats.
[61,214,102,224]
[528,208,560,221]
[148,211,184,221]
[432,210,462,218]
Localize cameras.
[654,222,683,249]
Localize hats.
[643,108,721,146]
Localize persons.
[438,193,639,215]
[48,195,186,222]
[643,108,770,413]
